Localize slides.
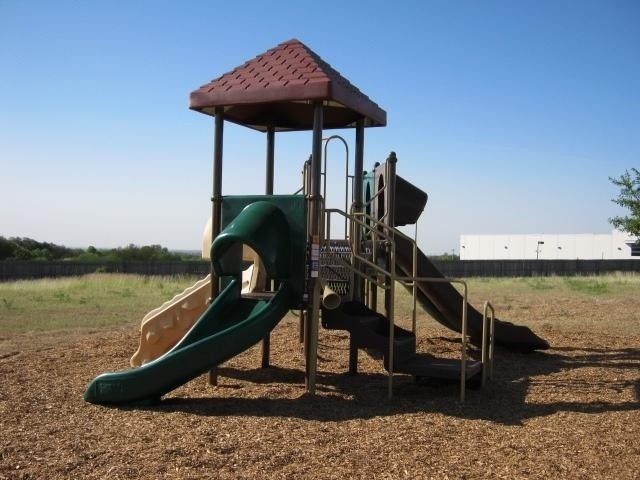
[392,227,550,352]
[130,272,213,368]
[84,276,293,406]
